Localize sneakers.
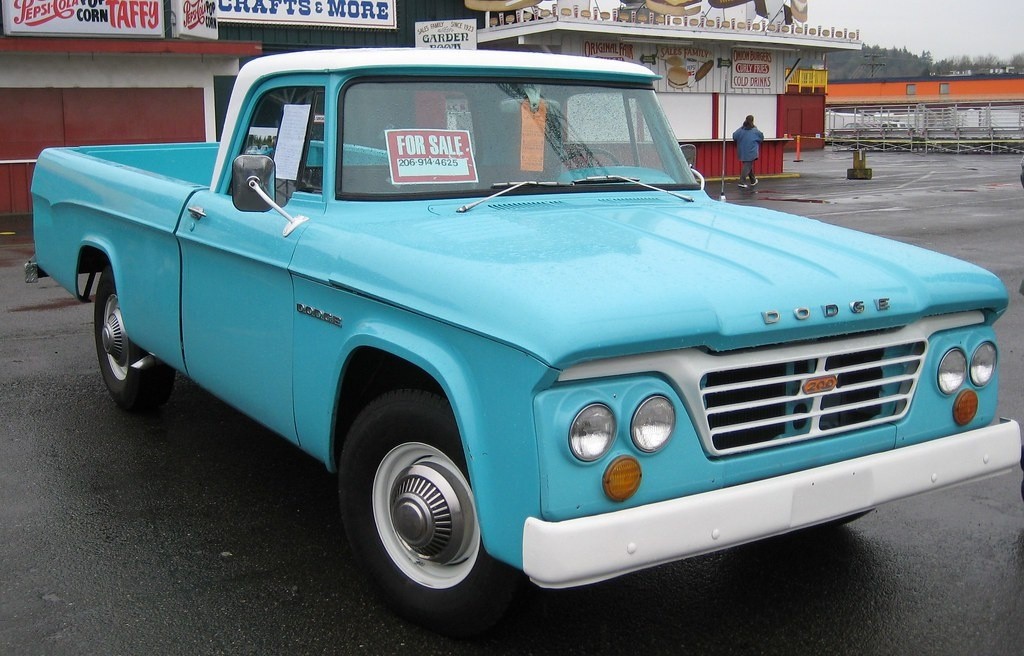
[751,179,758,185]
[738,183,747,187]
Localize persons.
[733,114,765,188]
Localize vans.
[880,120,913,130]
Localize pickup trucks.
[22,44,1024,632]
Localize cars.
[841,122,870,127]
